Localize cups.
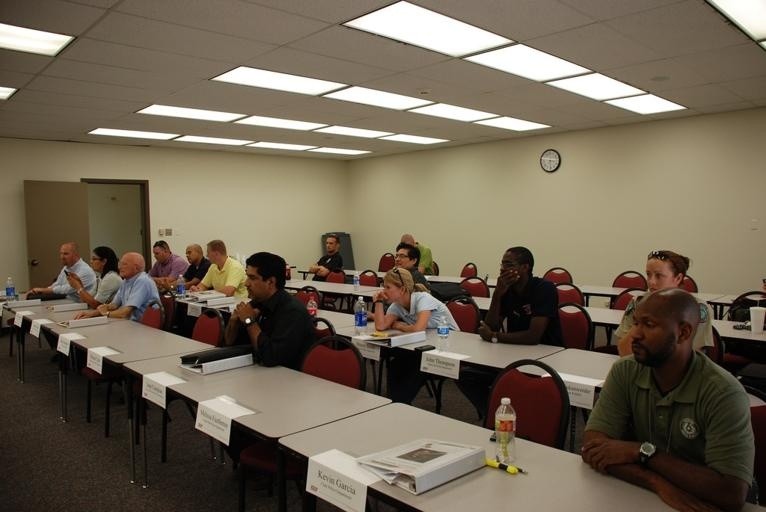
[750,305,766,334]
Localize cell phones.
[415,345,435,351]
[64,270,68,276]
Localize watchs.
[492,328,499,342]
[170,284,175,290]
[241,315,257,327]
[638,439,655,465]
[106,310,111,317]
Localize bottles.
[306,295,318,329]
[176,274,186,299]
[494,396,517,464]
[437,315,449,352]
[5,277,16,303]
[352,296,368,338]
[285,264,292,282]
[354,271,360,291]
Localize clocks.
[538,147,561,174]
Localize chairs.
[2,251,765,512]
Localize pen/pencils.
[484,459,526,474]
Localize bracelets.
[75,287,85,295]
[374,299,383,304]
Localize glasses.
[500,263,518,268]
[393,267,404,286]
[648,250,679,274]
[395,253,409,259]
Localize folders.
[2,299,41,308]
[46,303,88,312]
[365,331,426,349]
[57,316,109,328]
[180,344,254,375]
[190,290,235,306]
[355,438,486,495]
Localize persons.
[145,240,188,286]
[73,252,165,329]
[155,244,209,290]
[458,245,563,424]
[401,233,434,276]
[309,234,344,282]
[28,241,96,360]
[187,240,252,298]
[579,288,754,510]
[66,246,122,308]
[615,249,715,359]
[221,251,325,490]
[366,243,431,323]
[374,266,465,406]
